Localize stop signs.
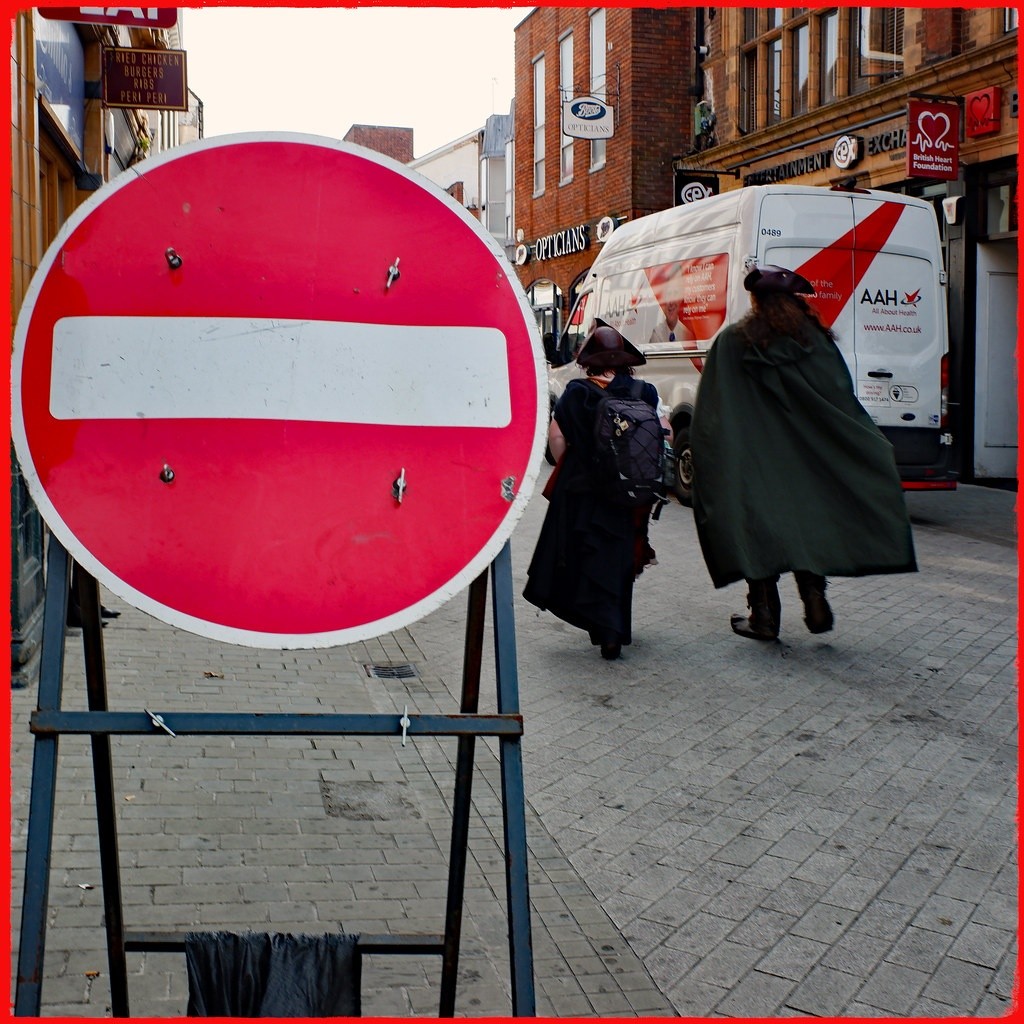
[10,128,551,654]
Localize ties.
[668,333,675,342]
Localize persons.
[67,588,122,627]
[522,318,674,660]
[689,265,920,641]
[649,263,694,344]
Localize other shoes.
[600,645,621,661]
[730,613,774,640]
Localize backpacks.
[566,378,668,508]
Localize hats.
[745,263,816,295]
[576,317,646,368]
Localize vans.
[542,185,962,492]
